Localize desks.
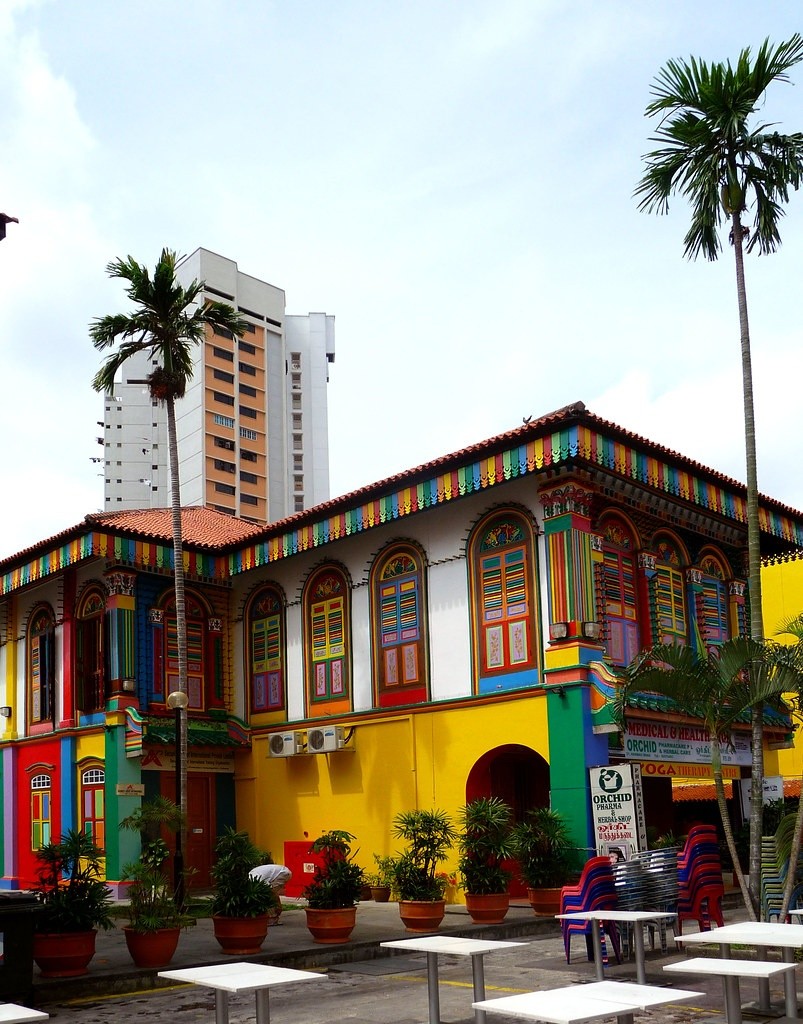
[158,962,278,1024]
[194,967,328,1024]
[595,910,678,988]
[555,911,630,985]
[546,980,707,1024]
[663,957,799,1024]
[714,921,803,1019]
[673,931,803,1023]
[430,940,530,1024]
[380,936,481,1024]
[472,991,640,1024]
[0,1002,49,1024]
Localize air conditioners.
[267,731,304,758]
[307,725,345,754]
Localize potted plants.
[369,853,393,902]
[117,794,200,968]
[29,827,117,980]
[201,825,281,956]
[385,808,461,933]
[302,830,372,944]
[359,875,372,901]
[460,796,521,925]
[514,806,569,918]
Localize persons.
[248,863,292,926]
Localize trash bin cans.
[0,893,45,1002]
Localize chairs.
[559,824,725,968]
[760,813,803,925]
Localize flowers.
[435,872,457,897]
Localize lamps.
[549,622,569,641]
[580,622,601,641]
[0,706,12,718]
[121,677,136,694]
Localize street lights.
[166,690,189,913]
